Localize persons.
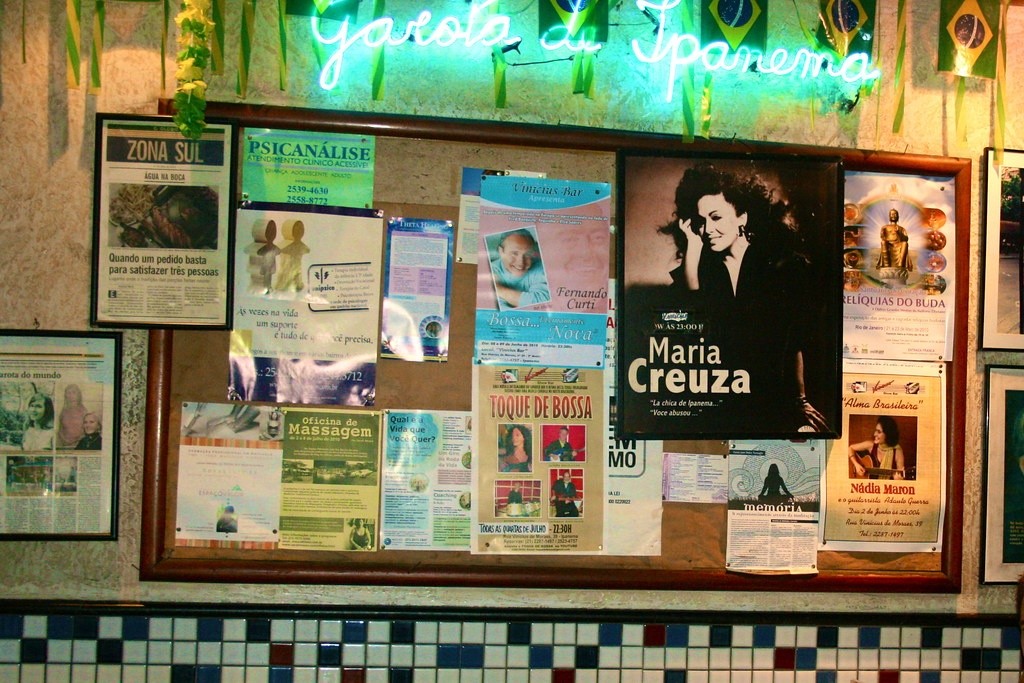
[490,229,551,308]
[507,480,526,517]
[427,323,441,337]
[848,417,907,481]
[21,383,102,450]
[551,473,579,517]
[545,427,579,461]
[878,207,912,270]
[498,424,533,473]
[665,166,831,435]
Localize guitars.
[498,458,532,472]
[545,444,585,462]
[550,491,584,507]
[847,449,917,481]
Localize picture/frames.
[90,112,240,332]
[979,148,1023,355]
[138,86,972,595]
[980,366,1024,585]
[1,329,123,541]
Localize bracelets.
[849,454,855,459]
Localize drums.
[496,501,540,516]
